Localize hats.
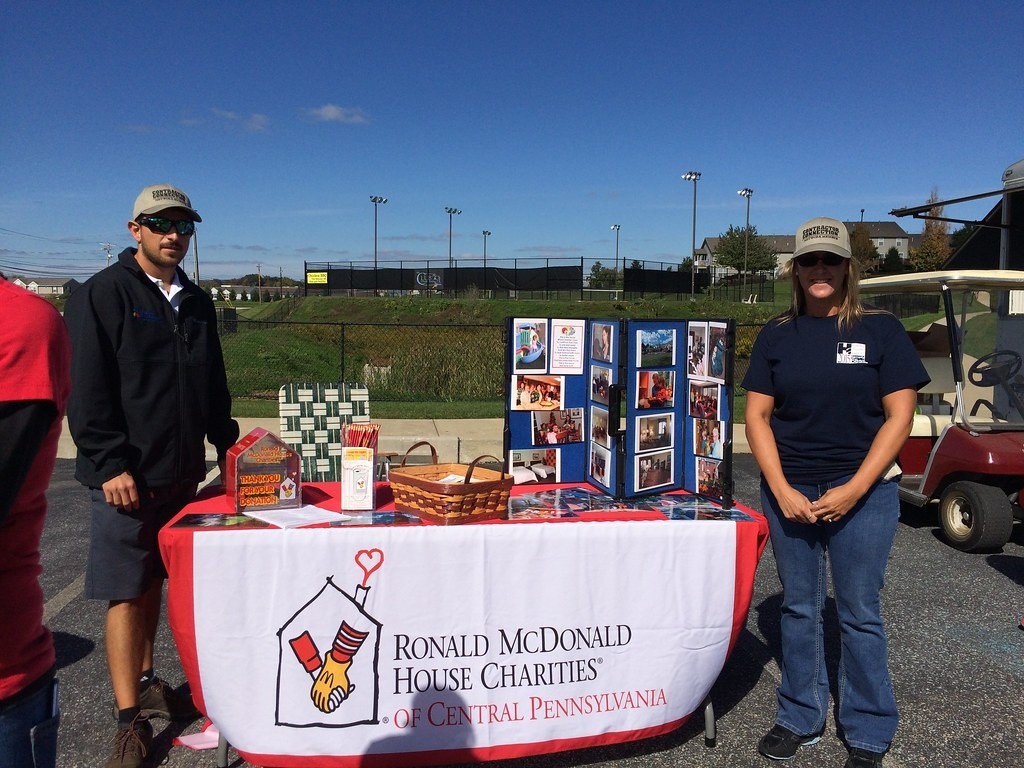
[132,183,204,226]
[790,215,853,260]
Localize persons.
[539,422,558,443]
[62,183,239,768]
[691,337,705,376]
[690,387,717,418]
[601,326,610,359]
[563,415,576,442]
[696,425,720,458]
[0,275,69,768]
[516,382,557,406]
[644,374,667,408]
[593,377,607,399]
[711,334,725,376]
[739,215,932,768]
[532,335,538,352]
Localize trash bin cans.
[216,308,237,337]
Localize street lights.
[480,230,491,298]
[611,224,622,287]
[681,171,703,301]
[738,188,753,297]
[444,206,462,292]
[369,195,388,296]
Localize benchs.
[578,289,624,302]
[909,356,1009,437]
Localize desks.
[158,484,771,768]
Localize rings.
[826,516,832,522]
[807,514,814,519]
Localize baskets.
[388,442,515,525]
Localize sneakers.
[104,707,154,768]
[758,723,821,760]
[138,673,201,721]
[842,748,883,768]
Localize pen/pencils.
[347,424,381,448]
[342,422,346,448]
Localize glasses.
[796,254,847,268]
[140,215,196,237]
